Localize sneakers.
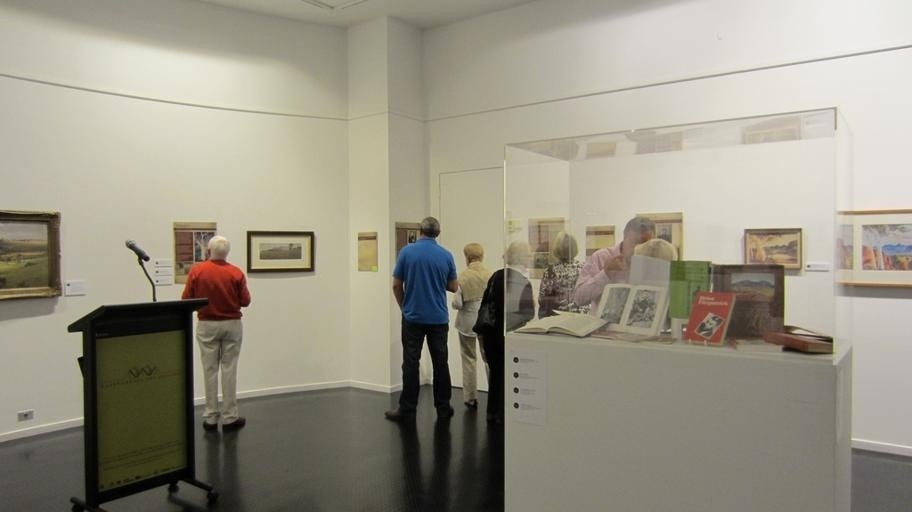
[464,399,478,417]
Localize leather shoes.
[202,420,231,436]
[383,405,417,422]
[223,416,247,431]
[437,404,456,419]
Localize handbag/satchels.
[472,272,499,334]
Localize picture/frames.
[836,208,912,286]
[246,230,314,274]
[742,228,801,271]
[2,210,60,297]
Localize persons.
[575,216,657,315]
[181,236,251,433]
[538,232,592,318]
[384,217,459,422]
[452,243,494,412]
[472,240,536,424]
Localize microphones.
[125,237,150,262]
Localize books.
[669,259,713,320]
[682,290,737,345]
[595,283,669,336]
[590,330,617,339]
[763,332,833,354]
[514,313,608,338]
[728,336,784,352]
